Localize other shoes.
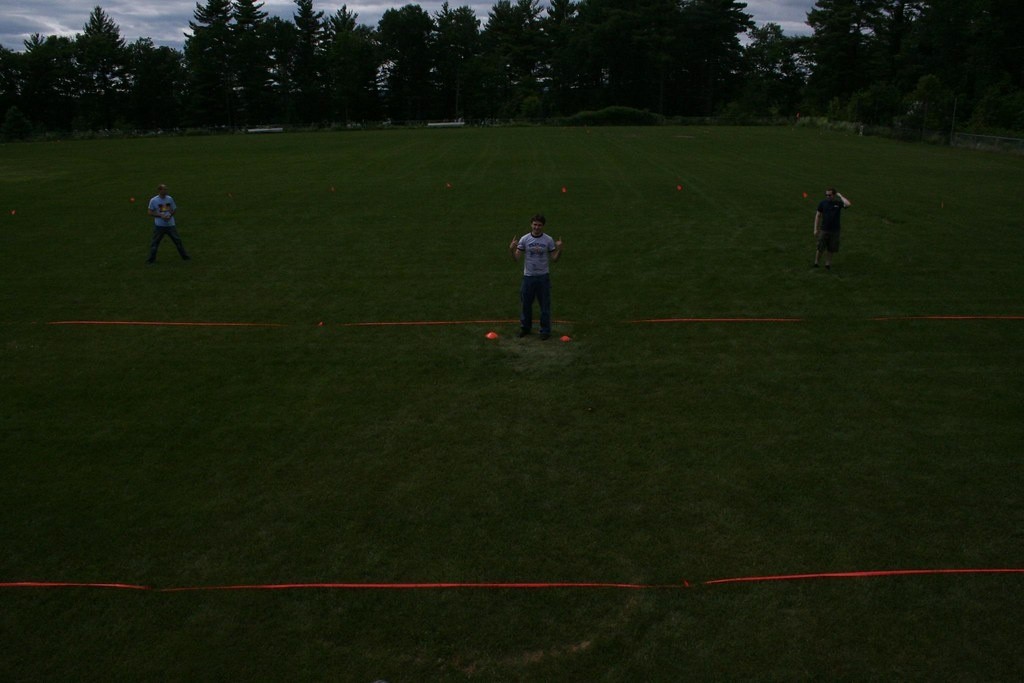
[541,334,549,340]
[517,331,528,337]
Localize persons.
[813,188,851,270]
[509,214,563,340]
[145,184,191,264]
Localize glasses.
[825,194,832,197]
